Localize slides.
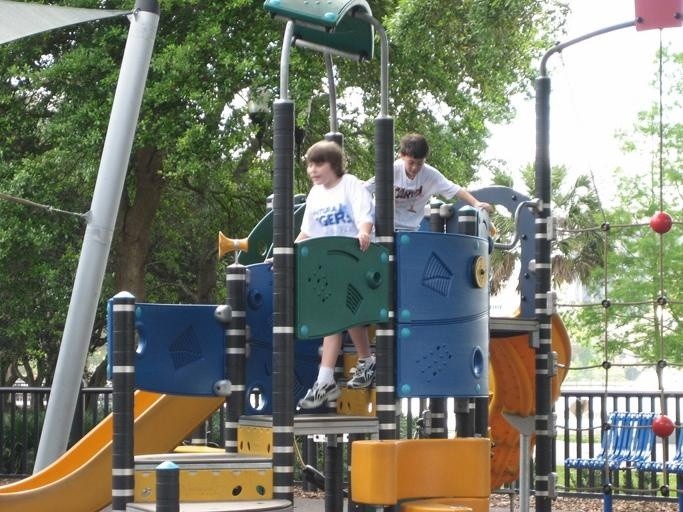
[488,311,569,494]
[1,389,227,512]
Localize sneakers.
[300,382,340,409]
[347,359,376,388]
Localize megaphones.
[218,231,249,258]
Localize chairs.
[563,412,683,512]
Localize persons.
[366,132,496,237]
[294,138,376,410]
